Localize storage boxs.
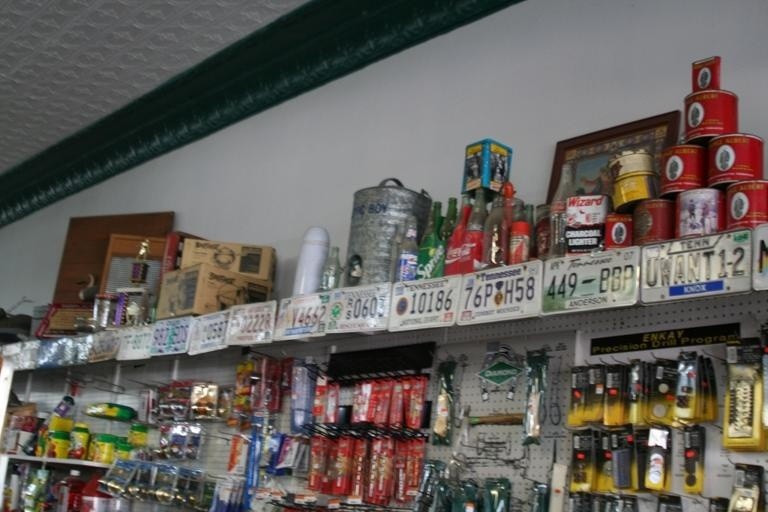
[156,263,271,321]
[181,237,275,281]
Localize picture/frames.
[545,111,680,205]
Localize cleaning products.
[4,468,83,512]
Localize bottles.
[395,184,547,282]
[547,163,578,262]
[319,246,343,293]
[293,226,329,297]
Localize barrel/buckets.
[344,178,431,286]
